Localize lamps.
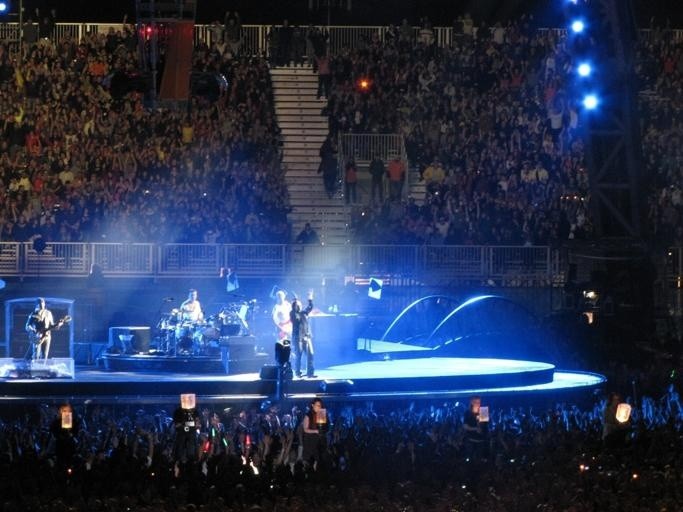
[479,406,489,432]
[181,393,196,427]
[316,408,327,433]
[61,411,73,433]
[615,403,631,423]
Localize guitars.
[28,315,72,345]
[277,309,319,338]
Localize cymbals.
[171,308,194,314]
[162,312,180,317]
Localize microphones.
[232,294,246,298]
[166,298,175,302]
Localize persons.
[0,16,681,511]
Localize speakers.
[259,364,293,379]
[107,327,150,352]
[319,380,355,393]
[221,347,254,359]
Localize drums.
[188,330,222,356]
[174,324,196,350]
[191,322,212,337]
